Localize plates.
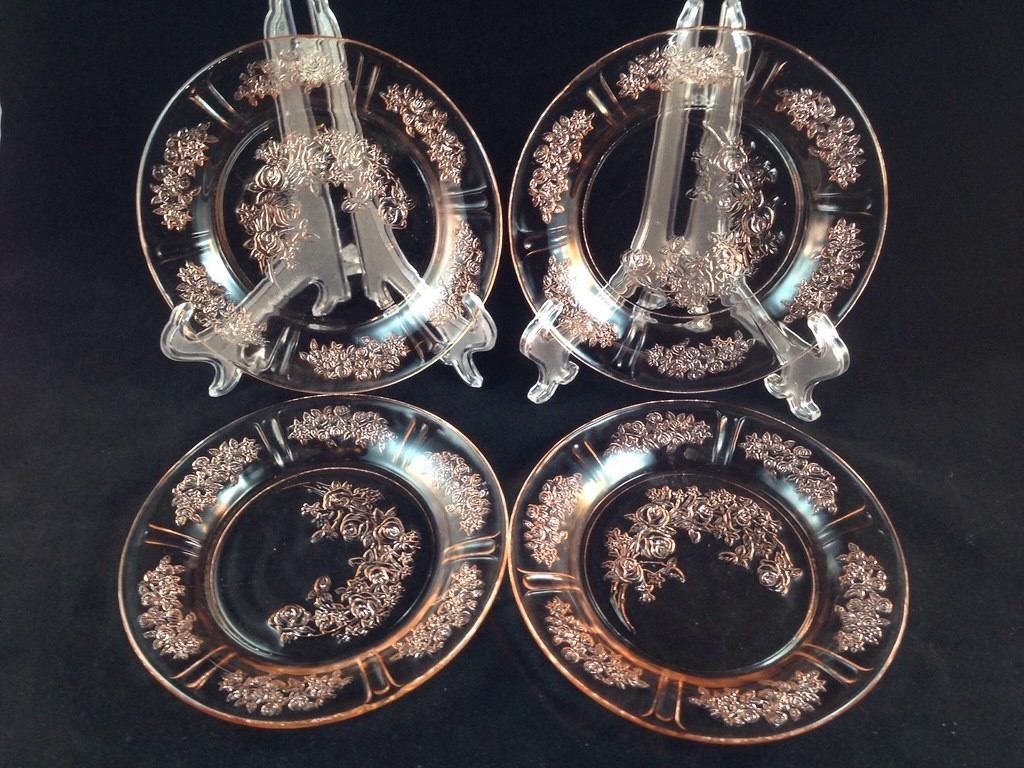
[508,27,888,391]
[139,37,503,394]
[119,395,509,726]
[508,399,910,745]
